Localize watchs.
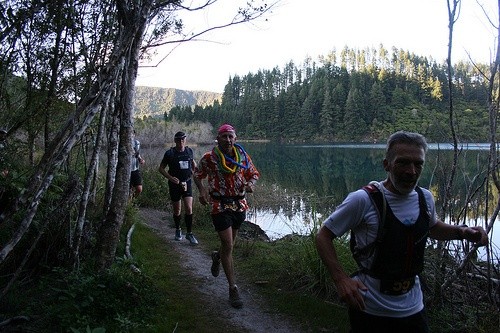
[458,224,468,237]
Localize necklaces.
[212,143,249,174]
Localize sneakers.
[186,233,198,244]
[176,229,183,240]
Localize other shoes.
[211,251,220,277]
[228,290,242,307]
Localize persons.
[193,124,260,307]
[159,131,199,245]
[315,132,488,333]
[130,130,145,206]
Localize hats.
[174,131,186,139]
[218,125,234,133]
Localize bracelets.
[250,181,253,184]
